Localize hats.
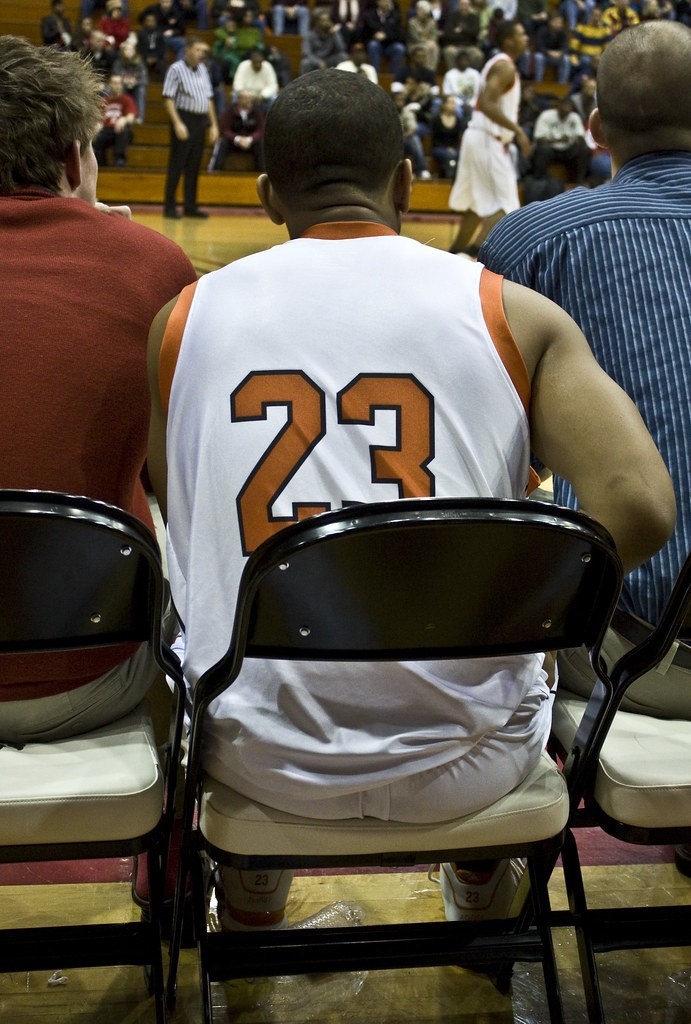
[106,0,123,12]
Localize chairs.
[0,487,197,1024]
[195,497,626,1024]
[562,553,691,1024]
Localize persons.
[35,0,691,254]
[149,69,679,930]
[475,20,691,723]
[0,31,197,912]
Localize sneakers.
[428,857,527,921]
[209,867,295,932]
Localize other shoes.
[421,170,431,179]
[113,157,125,166]
[184,207,208,217]
[390,81,403,93]
[164,209,181,218]
[132,852,149,908]
[675,844,691,878]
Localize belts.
[610,606,691,669]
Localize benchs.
[0,0,574,211]
